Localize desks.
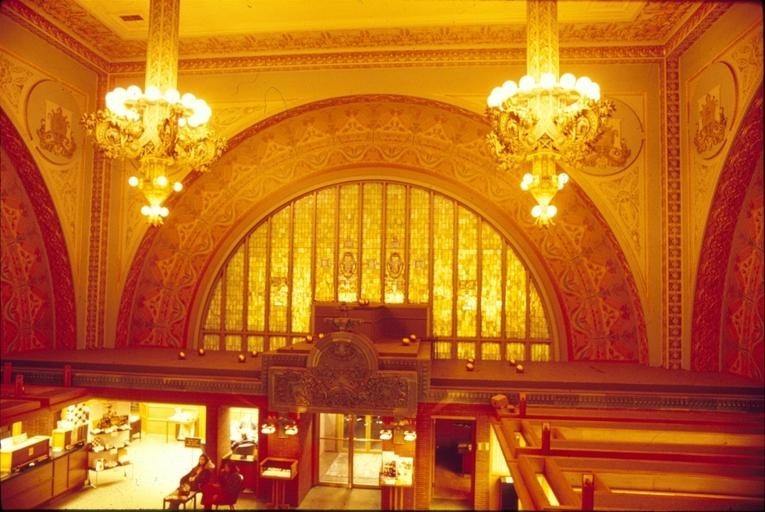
[164,486,197,510]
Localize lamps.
[486,2,602,228]
[83,2,226,230]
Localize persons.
[188,454,214,487]
[200,461,243,510]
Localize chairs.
[211,469,244,511]
